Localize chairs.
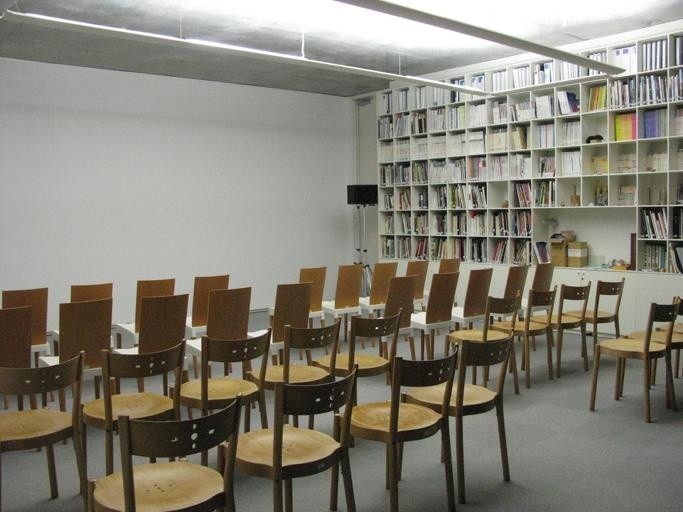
[330,345,460,510]
[485,262,557,394]
[87,391,237,511]
[394,332,511,503]
[589,301,680,423]
[590,296,682,410]
[564,278,625,371]
[215,363,357,511]
[531,280,591,379]
[0,253,520,501]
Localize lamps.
[0,0,625,97]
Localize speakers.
[347,184,377,204]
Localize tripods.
[354,204,373,297]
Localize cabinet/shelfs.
[377,19,682,275]
